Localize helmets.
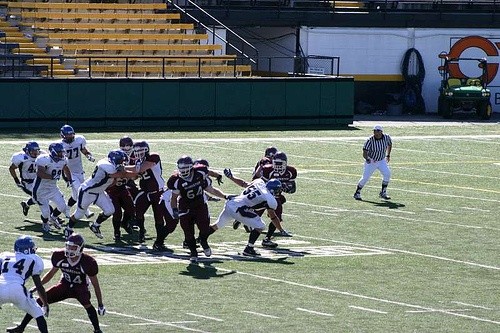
[60,125,75,143]
[266,178,282,198]
[25,142,40,158]
[14,236,35,254]
[65,233,84,252]
[49,143,65,161]
[108,136,209,170]
[265,147,277,158]
[273,152,287,162]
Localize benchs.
[0,0,262,78]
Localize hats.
[373,126,383,131]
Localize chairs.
[448,79,461,87]
[466,78,481,86]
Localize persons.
[167,157,239,261]
[6,235,105,333]
[353,126,392,200]
[0,236,49,333]
[104,138,179,253]
[32,143,72,233]
[62,151,139,240]
[9,142,66,224]
[196,146,297,257]
[61,125,95,218]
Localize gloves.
[51,174,60,180]
[280,230,292,236]
[85,152,96,163]
[224,195,238,200]
[172,208,180,217]
[215,175,224,186]
[98,304,105,316]
[224,169,232,179]
[208,197,220,202]
[16,182,24,188]
[42,304,49,317]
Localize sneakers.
[379,191,391,200]
[20,202,151,245]
[153,221,278,263]
[354,191,362,200]
[6,324,24,333]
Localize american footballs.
[282,183,293,193]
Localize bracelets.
[386,155,390,157]
